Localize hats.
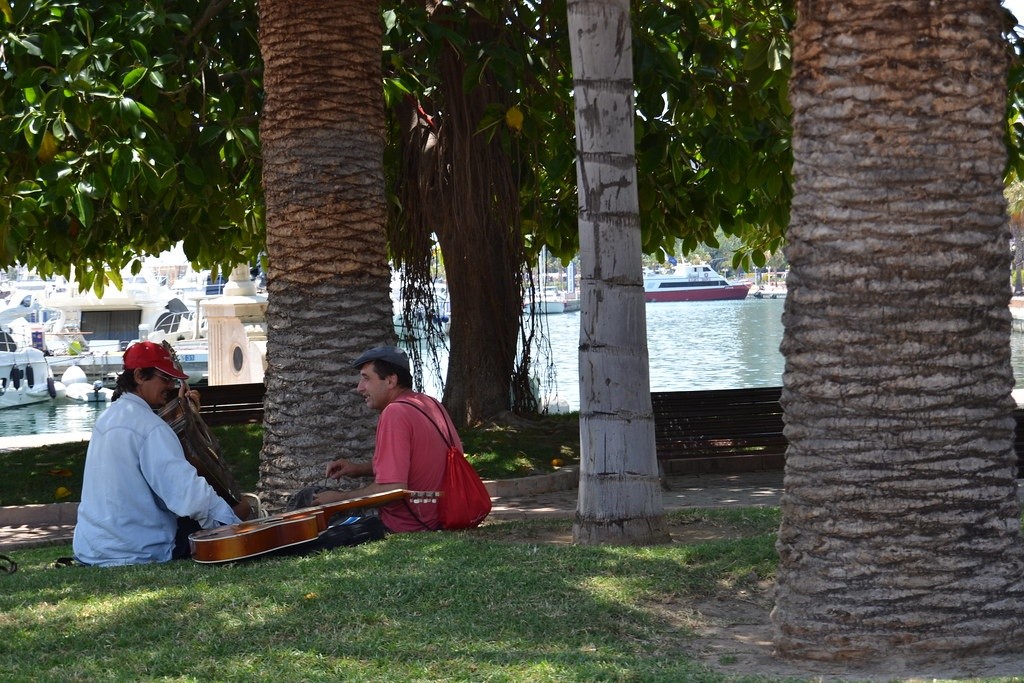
[351,346,410,371]
[122,341,190,381]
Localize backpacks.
[392,392,492,534]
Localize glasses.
[152,371,179,384]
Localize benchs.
[650,385,788,492]
[166,381,265,424]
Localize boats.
[748,284,787,299]
[388,261,451,341]
[645,252,753,302]
[0,239,267,409]
[523,286,581,314]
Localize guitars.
[157,339,243,507]
[187,488,444,563]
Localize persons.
[286,346,463,540]
[73,340,244,568]
